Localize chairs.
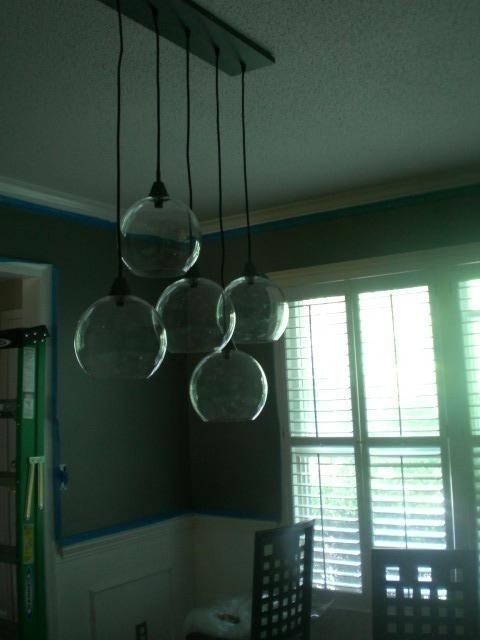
[250,519,315,640]
[371,546,478,638]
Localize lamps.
[120,5,195,277]
[73,0,166,384]
[154,9,237,354]
[216,39,291,341]
[188,31,270,423]
